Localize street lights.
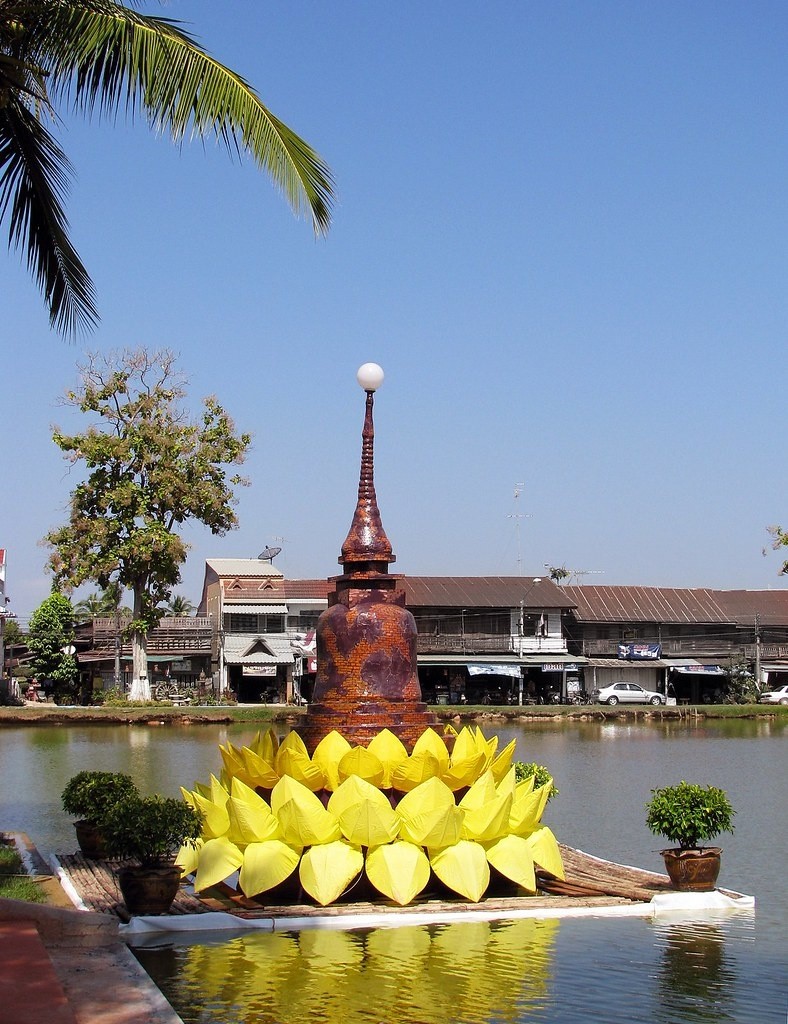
[518,577,542,708]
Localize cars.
[591,681,667,706]
[760,685,788,706]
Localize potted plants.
[61,770,141,861]
[644,779,738,893]
[93,792,206,916]
